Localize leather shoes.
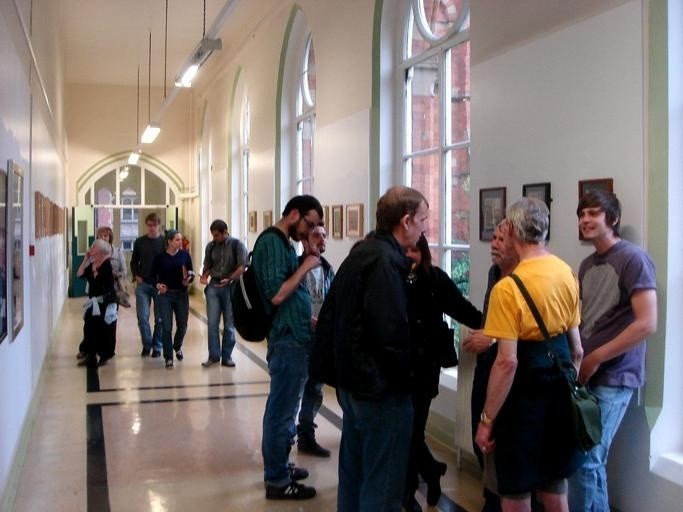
[200,357,220,367]
[424,461,448,507]
[141,347,149,357]
[150,350,161,358]
[220,359,235,367]
[402,494,422,512]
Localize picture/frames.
[247,210,257,233]
[261,210,272,232]
[4,157,26,343]
[344,202,363,238]
[520,182,551,241]
[477,185,507,243]
[33,189,65,243]
[330,204,343,240]
[576,177,614,241]
[321,205,329,237]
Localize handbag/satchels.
[553,378,605,456]
[228,225,291,344]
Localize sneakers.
[164,358,174,368]
[75,351,86,358]
[97,356,105,366]
[296,438,330,458]
[263,481,317,499]
[287,463,309,480]
[174,350,184,361]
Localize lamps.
[123,1,224,166]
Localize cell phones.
[87,251,90,256]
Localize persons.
[152,229,194,365]
[199,220,251,368]
[74,214,167,365]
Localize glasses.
[301,214,315,231]
